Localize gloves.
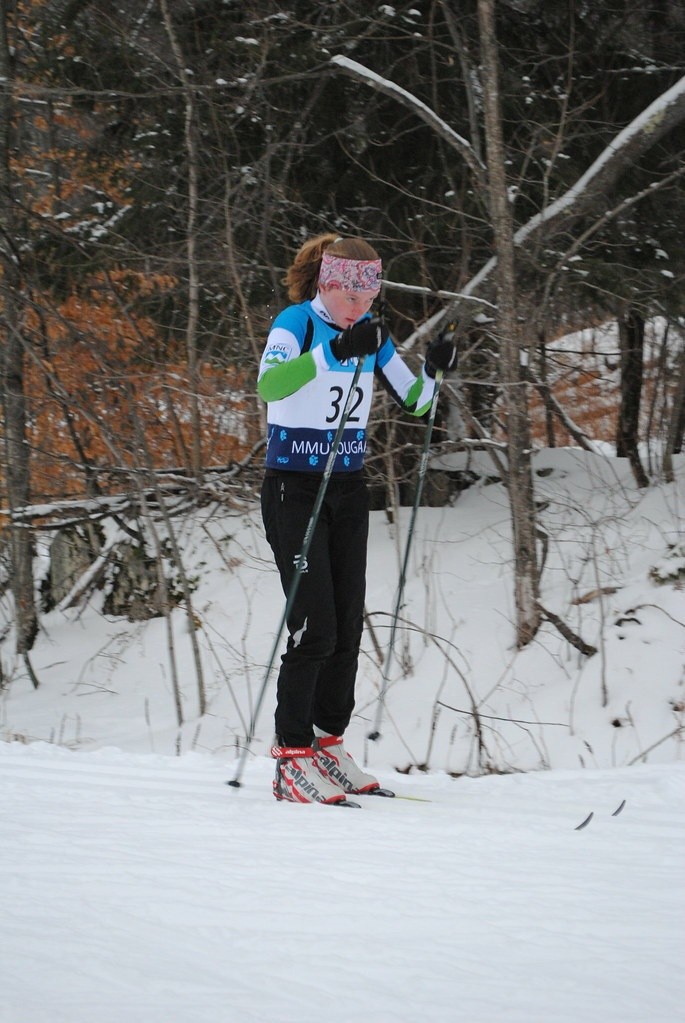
[329,317,389,360]
[422,332,458,381]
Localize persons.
[255,231,459,805]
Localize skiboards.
[332,795,628,833]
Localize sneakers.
[311,725,380,793]
[269,735,346,803]
